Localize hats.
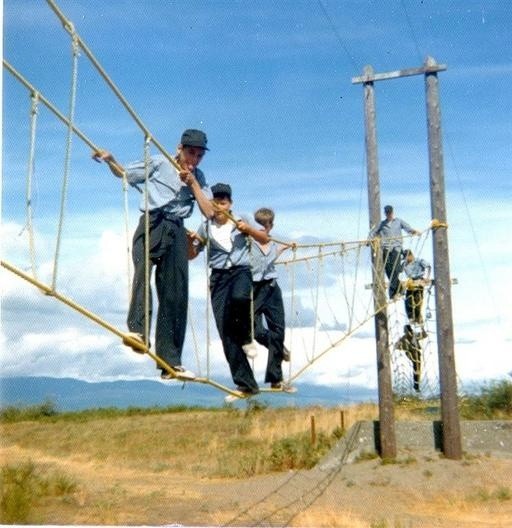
[179,128,212,154]
[209,182,232,198]
[401,249,411,261]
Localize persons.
[91,129,210,379]
[367,205,422,300]
[395,325,427,392]
[189,183,267,404]
[248,208,297,392]
[399,249,431,325]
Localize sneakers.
[123,332,152,356]
[224,389,256,404]
[270,380,298,394]
[281,345,292,363]
[392,292,405,302]
[242,339,258,358]
[161,365,197,382]
